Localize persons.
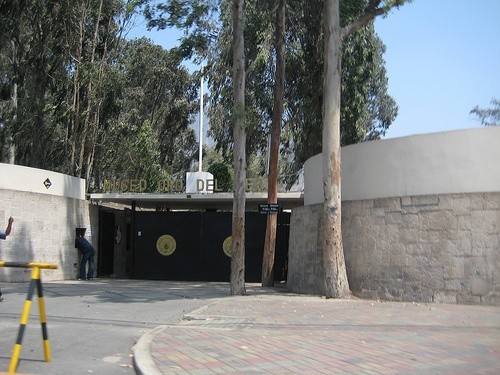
[0,217,15,302]
[74,237,95,281]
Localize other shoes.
[77,277,86,280]
[87,277,93,280]
[0,296,7,301]
